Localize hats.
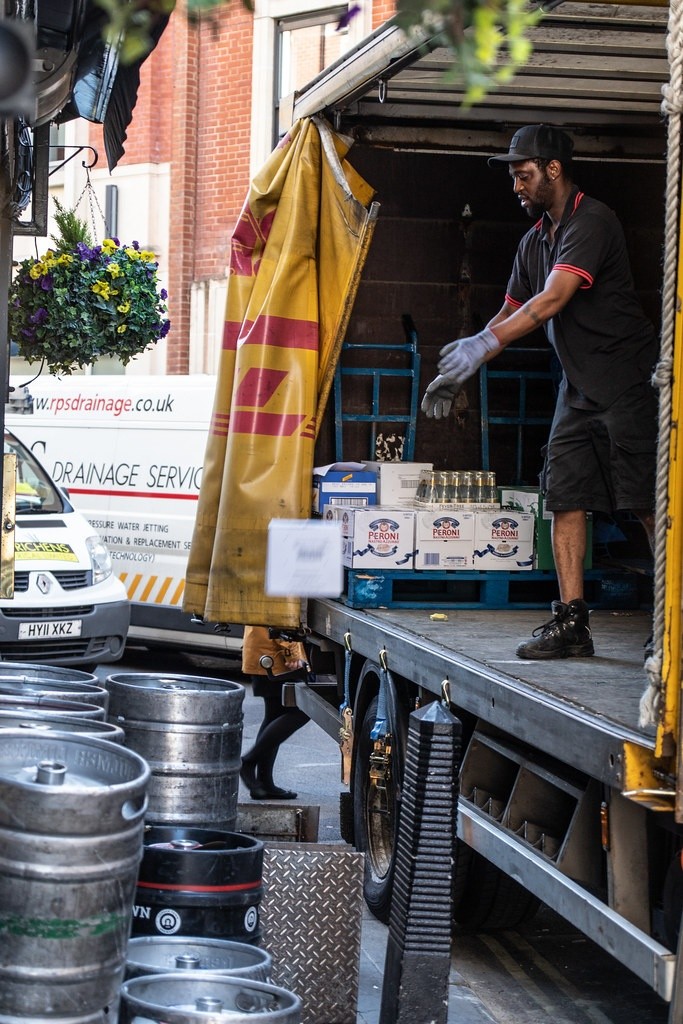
[487,122,574,167]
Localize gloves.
[421,376,461,420]
[436,329,501,382]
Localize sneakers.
[515,599,594,659]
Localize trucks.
[0,373,245,661]
[245,0,683,1024]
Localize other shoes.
[240,757,256,790]
[251,789,299,800]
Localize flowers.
[6,198,170,380]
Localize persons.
[420,123,659,663]
[234,624,317,800]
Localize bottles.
[414,469,499,503]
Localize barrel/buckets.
[1,661,302,1024]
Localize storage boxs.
[313,458,592,571]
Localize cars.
[0,422,131,675]
